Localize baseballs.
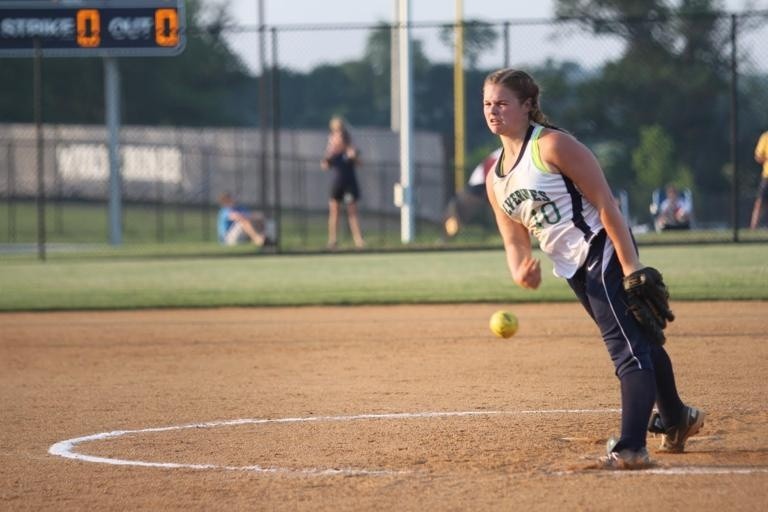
[489,311,520,339]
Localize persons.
[747,130,768,228]
[481,71,707,477]
[215,191,276,246]
[322,117,368,249]
[654,183,691,232]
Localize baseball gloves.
[624,267,674,346]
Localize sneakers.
[648,407,705,455]
[587,441,650,472]
